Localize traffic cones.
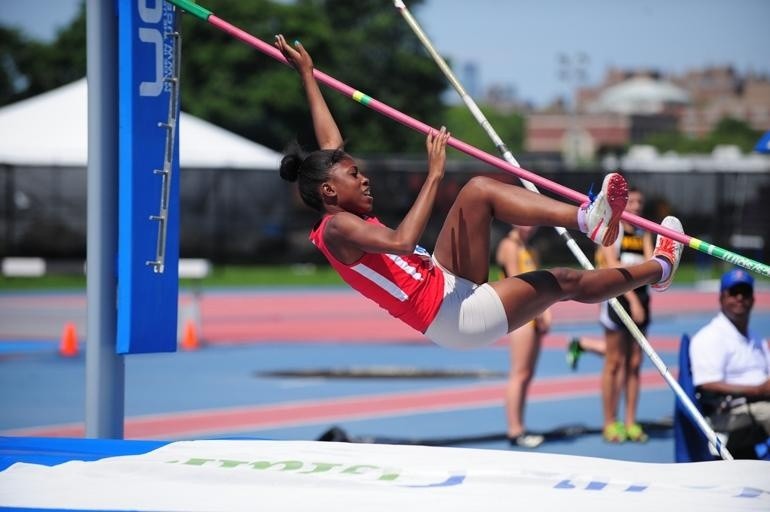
[59,321,81,357]
[179,320,198,350]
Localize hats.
[721,269,754,291]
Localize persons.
[496,220,551,449]
[689,269,770,438]
[273,32,685,350]
[566,333,610,368]
[593,183,656,443]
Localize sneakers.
[605,421,626,443]
[624,424,648,443]
[649,215,685,292]
[585,173,629,248]
[510,432,545,448]
[566,338,583,368]
